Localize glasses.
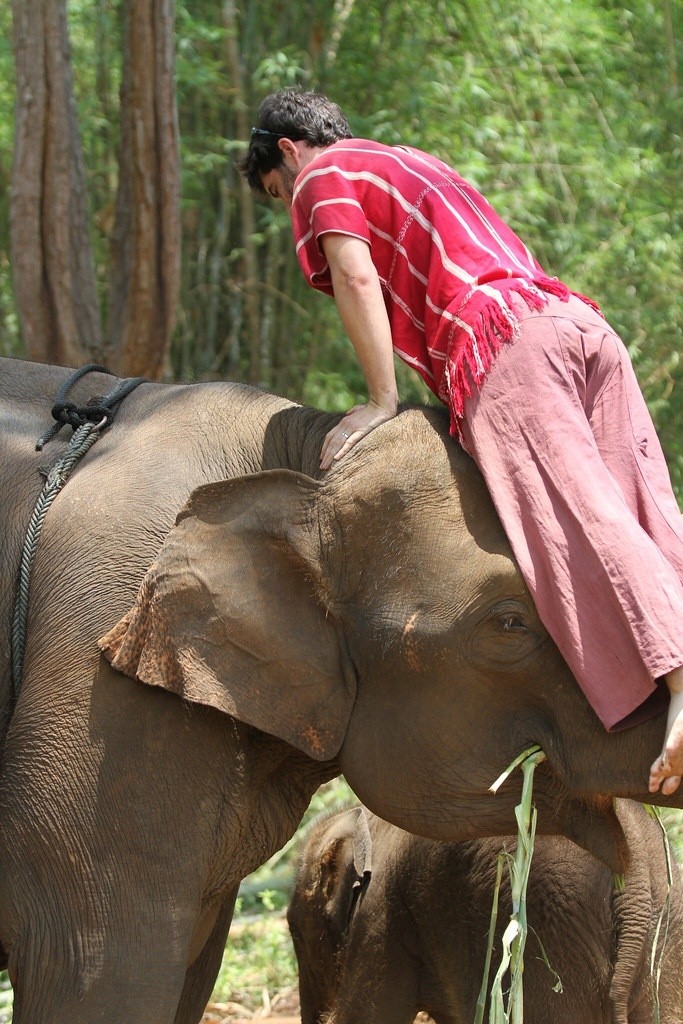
[249,126,288,150]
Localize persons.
[243,91,683,795]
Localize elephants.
[0,356,682,1022]
[286,805,682,1024]
